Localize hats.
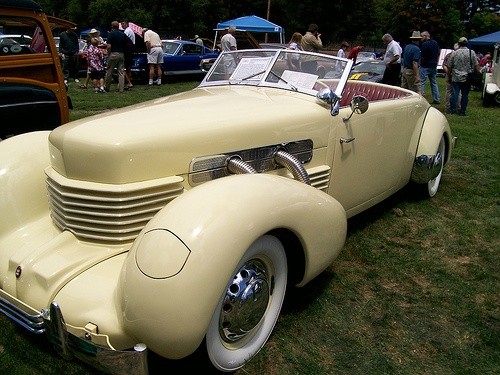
[458,37,469,43]
[141,28,147,36]
[410,30,423,39]
[88,28,101,36]
[194,35,200,39]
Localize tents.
[467,32,500,68]
[212,16,286,61]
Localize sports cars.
[323,59,388,83]
[0,48,458,375]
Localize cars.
[0,34,50,53]
[100,40,219,83]
[54,36,87,60]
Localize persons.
[142,27,164,85]
[60,20,129,91]
[194,35,204,46]
[121,19,136,88]
[419,31,441,103]
[301,24,323,73]
[448,37,478,115]
[335,41,349,73]
[348,43,365,60]
[470,50,492,91]
[444,42,463,109]
[379,34,403,86]
[285,32,303,71]
[373,51,385,60]
[221,25,241,79]
[402,31,422,95]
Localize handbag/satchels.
[467,70,483,85]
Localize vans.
[0,0,78,141]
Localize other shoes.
[74,79,80,83]
[429,100,442,105]
[78,84,88,88]
[115,88,125,92]
[458,113,467,117]
[128,84,135,89]
[105,86,113,92]
[156,81,162,86]
[452,111,458,114]
[63,80,69,86]
[94,87,99,93]
[101,89,107,93]
[148,82,154,86]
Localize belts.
[150,46,163,48]
